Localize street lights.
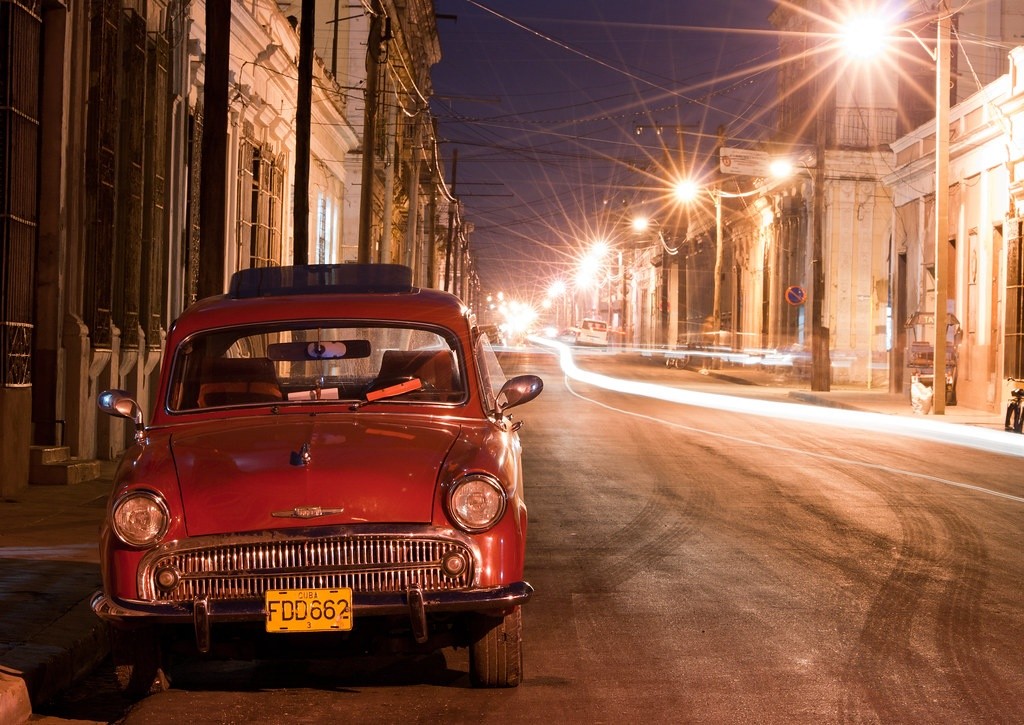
[592,242,627,354]
[841,13,947,415]
[674,180,720,370]
[633,215,668,349]
[771,156,822,391]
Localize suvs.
[89,261,544,690]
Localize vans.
[575,321,607,347]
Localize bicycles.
[666,348,690,370]
[1004,378,1024,434]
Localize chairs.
[198,357,281,409]
[370,350,461,404]
